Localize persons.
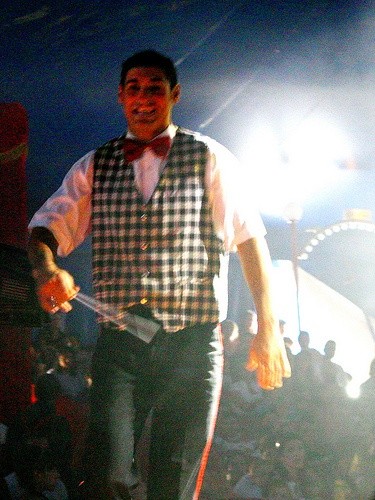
[0,308,375,500]
[27,51,291,500]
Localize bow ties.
[123,135,172,162]
